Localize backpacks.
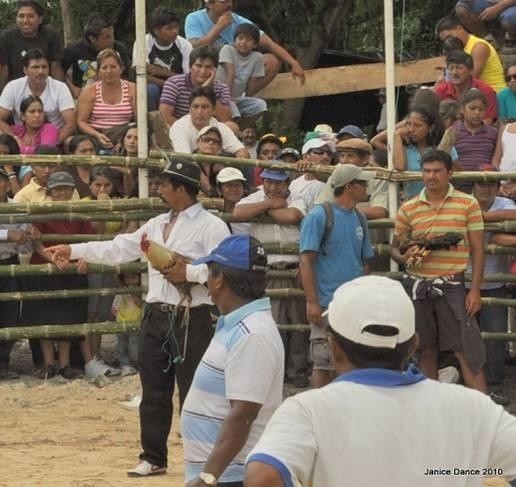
[317,201,367,252]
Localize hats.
[305,123,339,142]
[336,125,365,139]
[321,276,415,347]
[215,167,248,182]
[276,147,300,160]
[301,138,336,156]
[197,125,222,142]
[193,232,270,271]
[164,159,203,187]
[329,164,376,189]
[336,139,372,154]
[260,159,292,180]
[262,133,288,146]
[45,172,80,193]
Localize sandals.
[34,363,57,379]
[60,365,84,379]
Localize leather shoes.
[0,368,19,379]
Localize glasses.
[201,136,222,145]
[506,73,516,81]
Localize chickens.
[393,227,465,270]
[140,232,208,326]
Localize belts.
[153,303,187,312]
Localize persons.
[298,164,378,389]
[184,0,306,98]
[392,106,458,200]
[160,45,239,137]
[170,87,251,161]
[0,134,34,196]
[131,6,195,112]
[246,276,515,487]
[0,48,75,146]
[80,164,127,379]
[370,88,445,150]
[495,62,515,122]
[11,95,57,163]
[108,121,152,197]
[433,54,497,126]
[78,48,136,147]
[324,137,387,220]
[39,154,233,475]
[216,168,245,212]
[181,235,285,487]
[12,146,81,206]
[231,159,310,350]
[438,88,497,193]
[66,13,132,97]
[130,169,164,231]
[389,148,487,393]
[460,162,516,406]
[112,263,146,374]
[303,124,367,142]
[0,168,29,380]
[240,123,259,159]
[288,139,336,209]
[435,16,509,92]
[256,134,300,176]
[189,126,229,197]
[217,23,267,130]
[30,171,93,379]
[491,120,516,202]
[456,1,516,49]
[1,1,63,90]
[65,135,101,198]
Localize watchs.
[198,469,219,486]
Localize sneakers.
[293,372,311,388]
[122,365,135,376]
[492,391,510,404]
[84,358,122,377]
[128,460,167,476]
